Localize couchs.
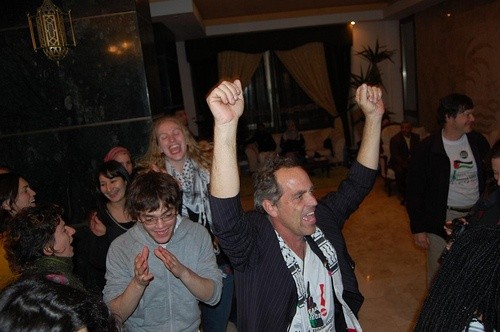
[244,127,345,177]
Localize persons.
[144,116,238,332]
[0,146,139,332]
[170,105,199,143]
[279,118,307,166]
[206,78,384,332]
[417,223,500,332]
[445,135,500,233]
[248,122,277,170]
[391,119,420,204]
[405,93,491,286]
[103,171,223,332]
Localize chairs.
[380,124,424,197]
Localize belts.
[447,205,474,213]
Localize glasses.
[138,208,177,224]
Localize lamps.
[27,0,76,66]
